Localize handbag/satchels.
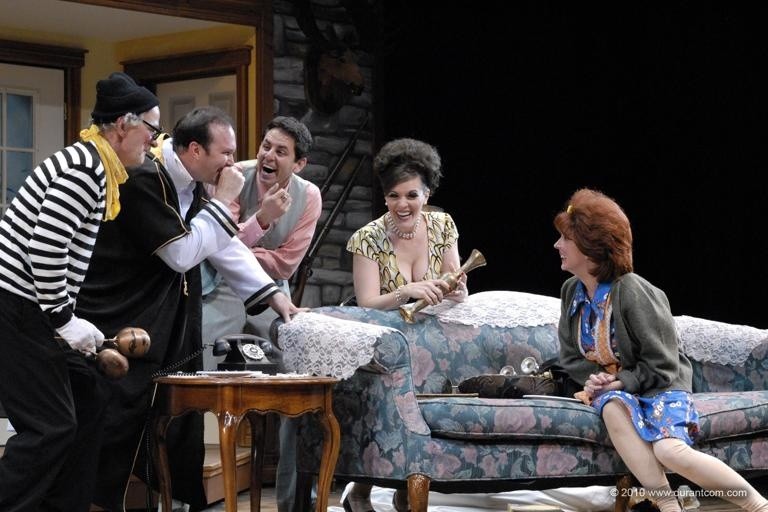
[458,375,554,398]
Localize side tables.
[154,374,341,512]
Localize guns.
[288,115,369,309]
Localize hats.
[91,72,160,125]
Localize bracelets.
[394,285,411,306]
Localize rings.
[280,194,286,202]
[587,384,593,387]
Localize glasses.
[143,120,161,140]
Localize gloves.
[55,313,105,358]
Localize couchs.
[269,290,768,512]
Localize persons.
[339,136,468,511]
[0,73,164,511]
[552,188,768,511]
[74,104,313,512]
[201,115,322,510]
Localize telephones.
[212,333,282,375]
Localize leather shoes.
[342,492,376,512]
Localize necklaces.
[385,212,423,239]
